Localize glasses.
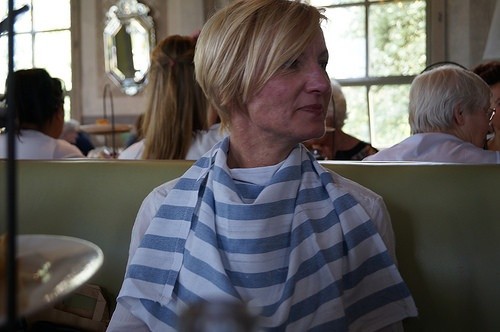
[464,104,497,123]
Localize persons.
[0,68,87,159]
[60,119,80,144]
[301,78,378,161]
[420,60,467,74]
[123,114,148,149]
[106,0,418,332]
[87,147,113,159]
[71,130,94,156]
[118,34,232,160]
[473,60,500,153]
[361,64,500,163]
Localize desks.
[80,124,132,148]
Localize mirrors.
[102,1,156,96]
